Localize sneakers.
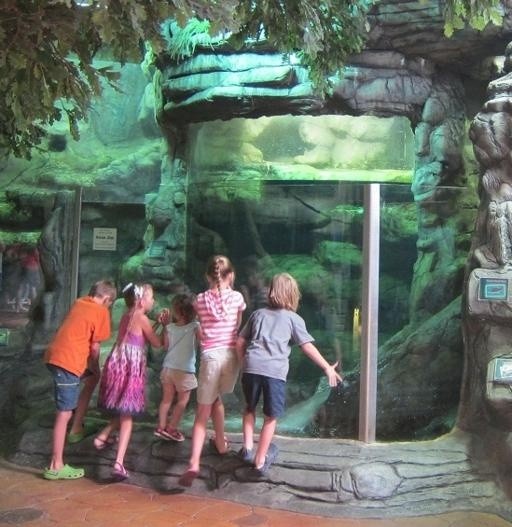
[152,424,185,443]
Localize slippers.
[210,434,231,455]
[177,470,198,488]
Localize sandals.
[238,440,282,475]
[43,463,85,482]
[67,420,121,451]
[110,462,129,480]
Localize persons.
[154,294,202,442]
[93,283,169,478]
[179,255,248,487]
[236,272,343,477]
[43,279,116,480]
